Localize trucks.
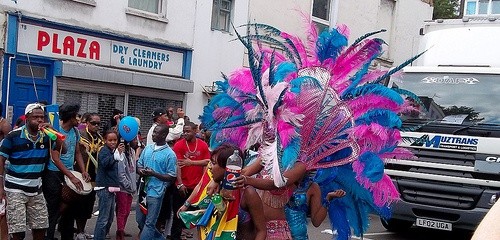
[373,14,500,235]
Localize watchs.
[177,184,184,189]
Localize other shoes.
[73,232,94,240]
[116,230,134,240]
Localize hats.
[148,108,169,123]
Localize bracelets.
[320,199,331,209]
[183,201,191,208]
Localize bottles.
[211,193,226,214]
[223,150,242,199]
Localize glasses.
[87,119,103,127]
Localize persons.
[0,4,432,240]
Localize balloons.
[119,116,139,141]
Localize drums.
[58,170,92,212]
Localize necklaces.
[184,138,198,154]
